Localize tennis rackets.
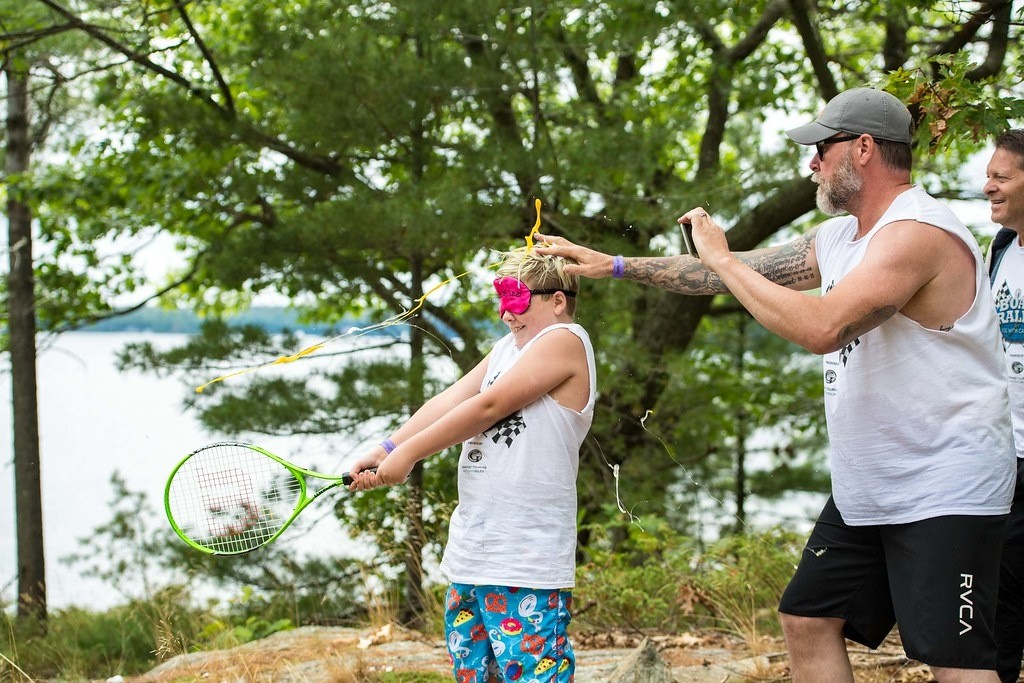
[163,442,412,556]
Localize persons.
[534,85,1024,683]
[346,244,598,682]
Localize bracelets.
[612,255,624,279]
[379,439,396,454]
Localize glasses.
[815,135,881,161]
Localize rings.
[700,212,707,217]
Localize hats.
[785,88,914,145]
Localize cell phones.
[680,222,701,259]
